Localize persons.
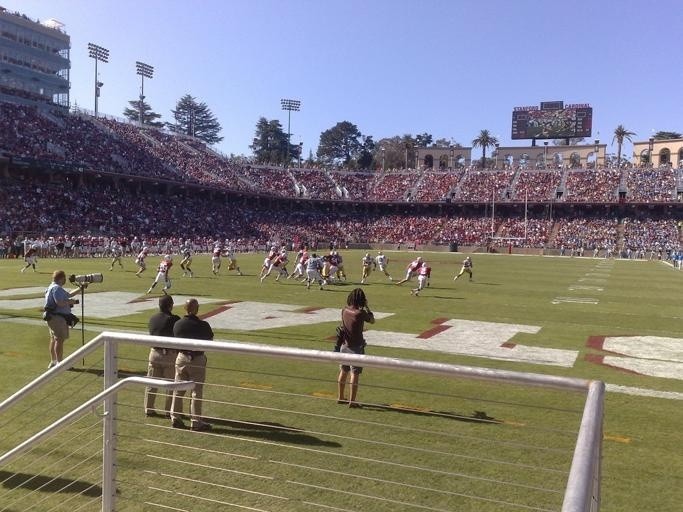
[171,299,214,430]
[43,271,85,370]
[144,295,182,418]
[336,288,374,409]
[0,96,683,295]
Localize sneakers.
[21,270,23,272]
[391,277,393,280]
[469,280,472,281]
[312,276,346,289]
[411,289,418,296]
[454,276,457,280]
[191,424,210,431]
[349,404,362,408]
[34,271,35,272]
[172,422,184,428]
[336,399,348,404]
[212,270,218,275]
[259,274,263,282]
[237,266,242,275]
[396,283,401,286]
[361,280,363,283]
[109,269,112,271]
[162,289,166,294]
[145,411,156,417]
[48,362,73,370]
[287,276,310,289]
[135,274,141,278]
[147,291,148,294]
[165,414,169,419]
[182,272,193,277]
[276,275,279,282]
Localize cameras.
[68,273,103,287]
[69,299,79,307]
[332,326,346,352]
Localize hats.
[467,256,471,260]
[304,252,308,256]
[366,253,371,258]
[312,254,317,257]
[378,252,382,255]
[423,263,427,267]
[417,257,422,260]
[330,251,339,256]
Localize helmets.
[33,245,37,250]
[165,256,172,261]
[180,245,184,250]
[272,246,286,253]
[143,247,149,252]
